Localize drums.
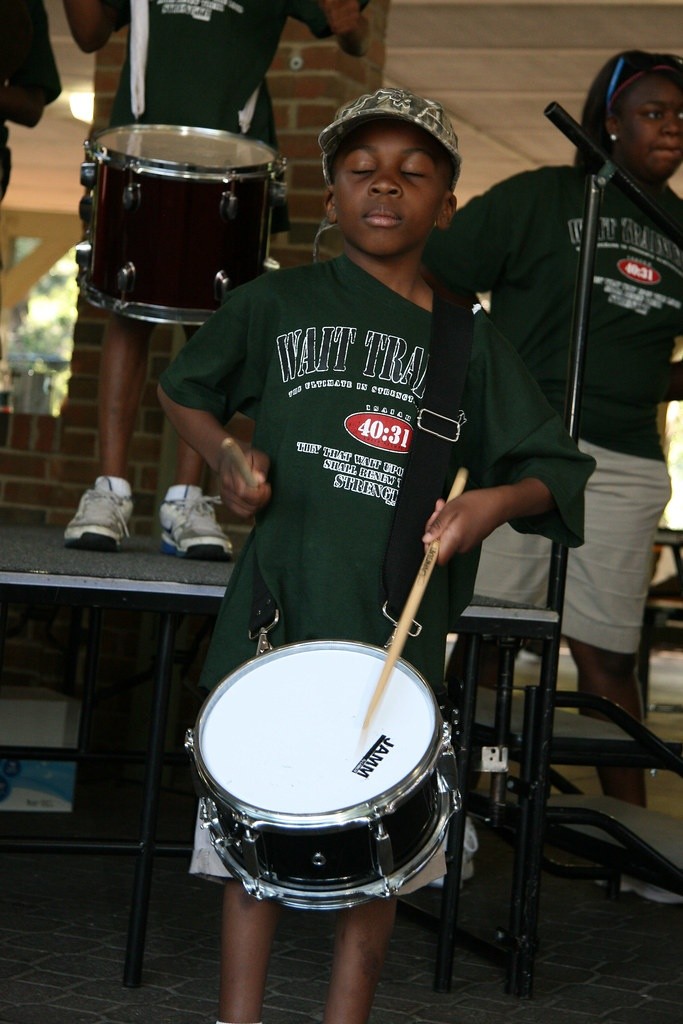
[185,636,465,913]
[73,122,289,330]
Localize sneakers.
[63,489,134,554]
[159,496,234,562]
[594,863,683,904]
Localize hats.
[318,88,462,194]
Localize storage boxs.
[1,685,82,813]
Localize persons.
[0,1,62,204]
[46,0,379,558]
[152,80,592,1023]
[451,48,683,911]
